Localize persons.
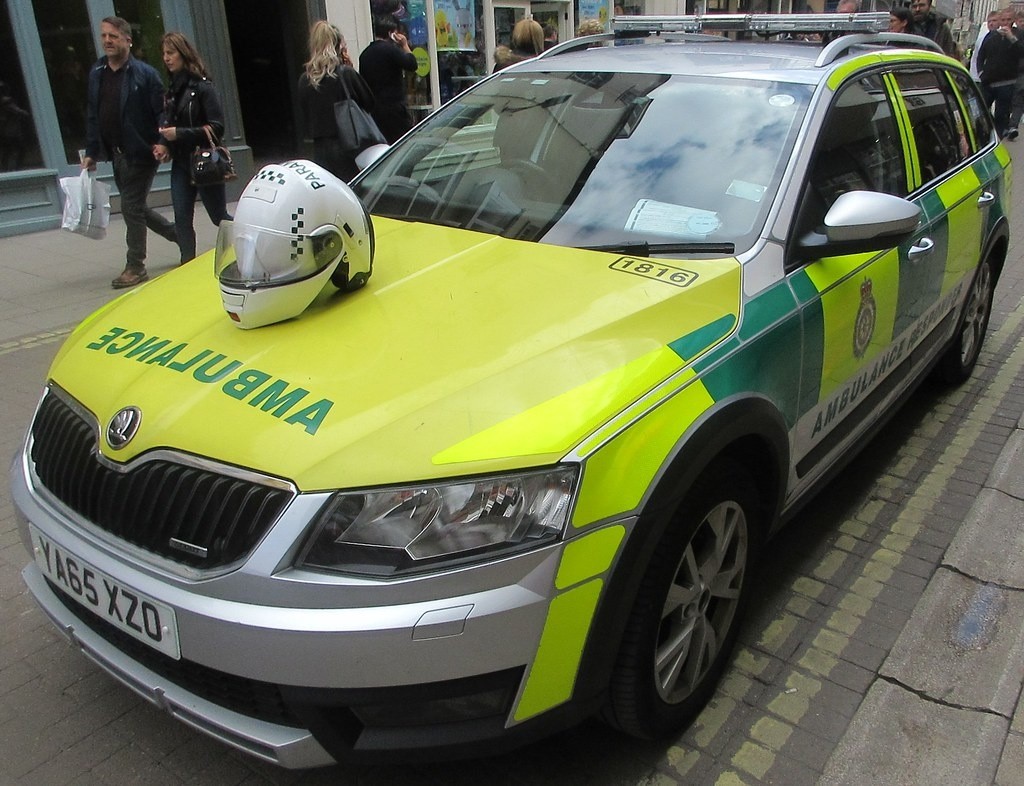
[298,21,377,184]
[360,16,419,141]
[494,17,608,161]
[79,16,235,288]
[832,0,1024,141]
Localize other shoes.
[1007,127,1018,142]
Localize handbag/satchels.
[189,125,238,185]
[332,66,388,156]
[58,167,111,242]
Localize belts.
[108,146,122,153]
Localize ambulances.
[10,12,1011,771]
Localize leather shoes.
[111,264,148,289]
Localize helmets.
[214,161,376,332]
